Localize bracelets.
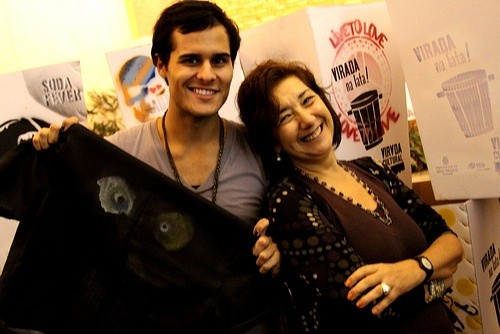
[425,278,446,304]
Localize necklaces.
[162,109,225,203]
[292,161,392,227]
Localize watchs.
[411,255,433,286]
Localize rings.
[381,282,392,295]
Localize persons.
[236,58,464,334]
[33,1,283,334]
